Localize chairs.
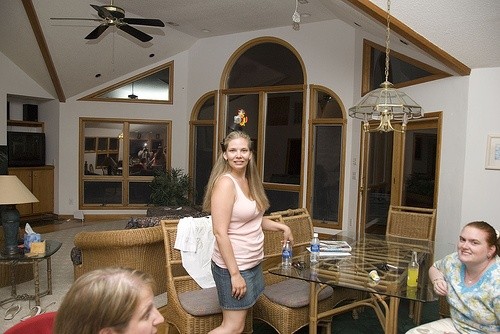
[271,208,359,320]
[253,215,334,334]
[385,205,437,241]
[160,219,253,334]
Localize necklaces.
[466,260,490,283]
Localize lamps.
[348,0,424,134]
[0,175,39,254]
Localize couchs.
[70,217,189,297]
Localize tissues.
[24,223,41,250]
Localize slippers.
[4,303,20,320]
[20,306,46,321]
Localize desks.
[268,229,459,334]
[0,240,61,306]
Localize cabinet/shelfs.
[8,165,54,217]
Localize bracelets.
[432,278,444,284]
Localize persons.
[52,264,164,334]
[404,220,500,334]
[149,150,164,175]
[202,131,295,334]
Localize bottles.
[310,263,319,280]
[84,161,88,174]
[281,241,292,268]
[407,251,419,288]
[89,164,93,174]
[407,286,417,299]
[309,232,320,262]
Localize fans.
[49,0,165,42]
